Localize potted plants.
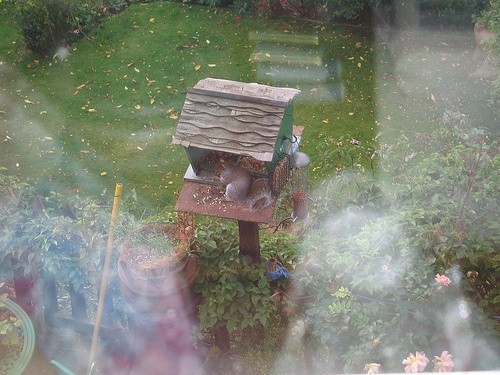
[1,283,37,375]
[102,210,191,278]
[470,7,500,61]
